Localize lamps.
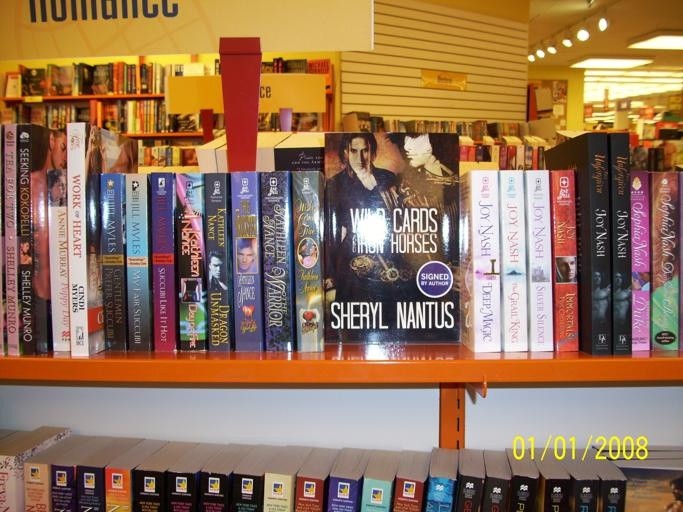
[527,8,609,64]
[568,55,653,69]
[626,31,683,50]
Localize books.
[1,427,682,511]
[2,59,333,134]
[1,110,683,357]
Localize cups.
[277,107,293,131]
